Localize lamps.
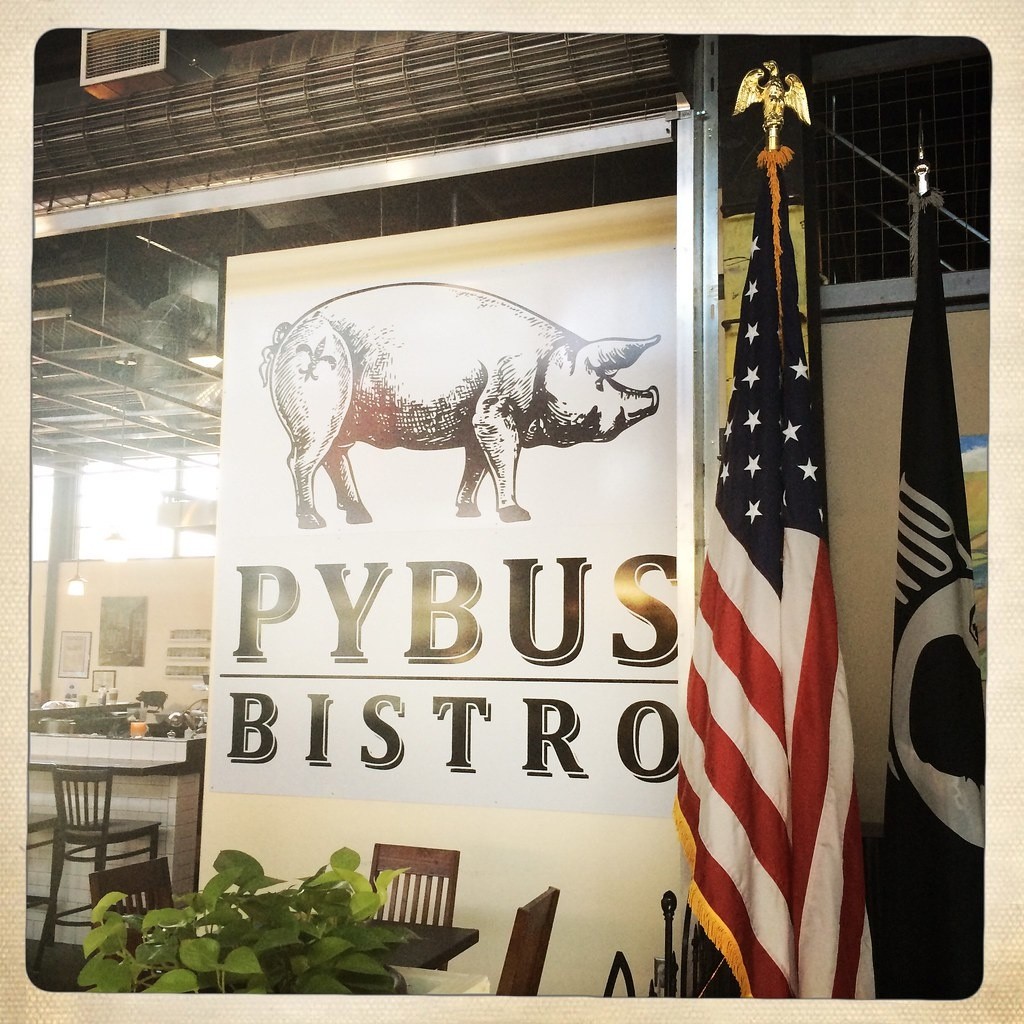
[66,528,86,595]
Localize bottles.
[98,685,106,706]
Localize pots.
[39,718,76,734]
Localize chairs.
[498,886,561,999]
[371,842,461,971]
[87,858,175,964]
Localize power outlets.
[654,957,666,989]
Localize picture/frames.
[92,671,117,695]
[56,631,93,679]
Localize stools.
[26,763,162,974]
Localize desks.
[367,918,480,968]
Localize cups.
[78,695,87,706]
[131,722,149,740]
[110,689,118,704]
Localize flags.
[879,189,986,1000]
[672,154,875,1000]
